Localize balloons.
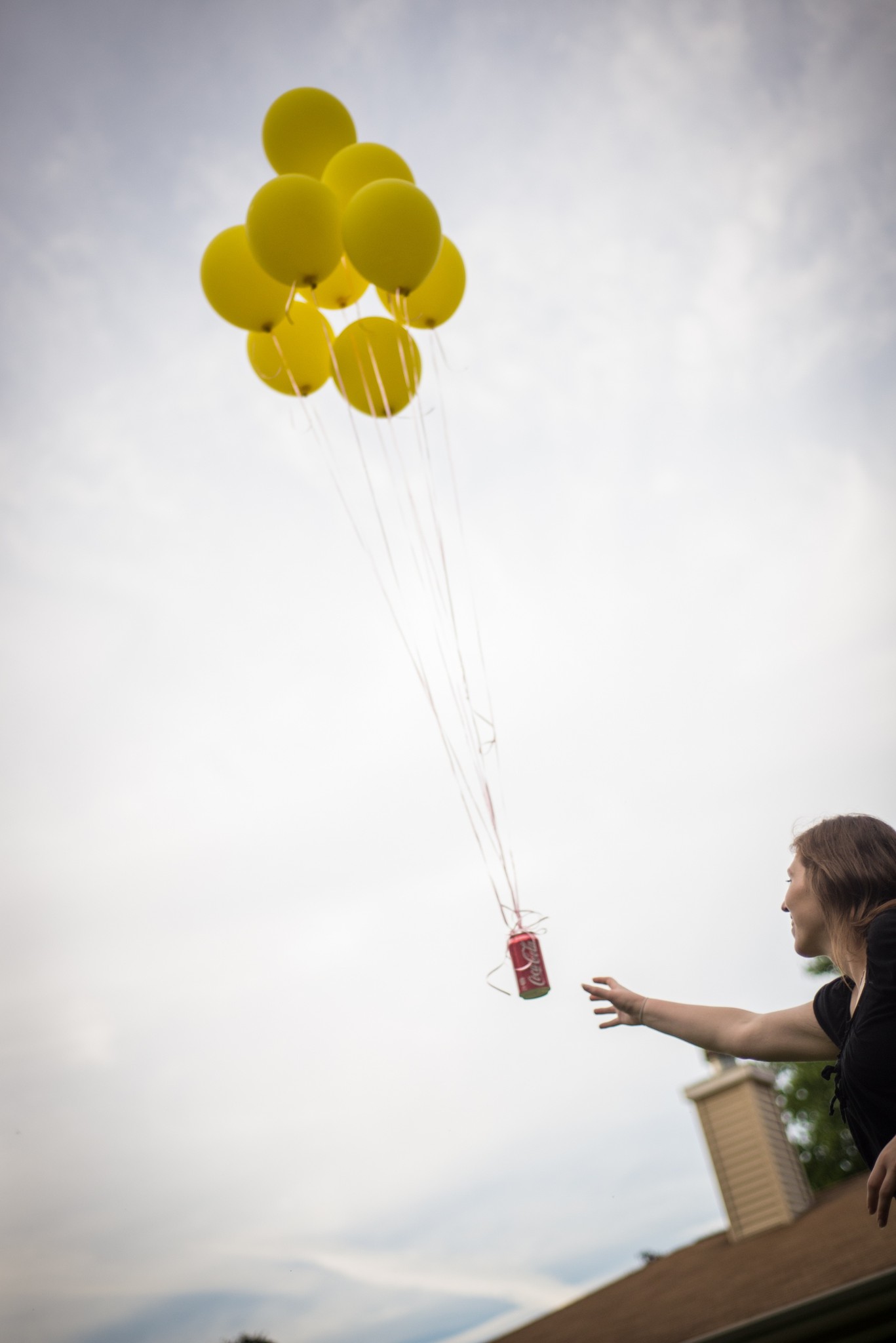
[201,86,466,422]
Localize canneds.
[508,931,550,999]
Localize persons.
[577,813,896,1232]
